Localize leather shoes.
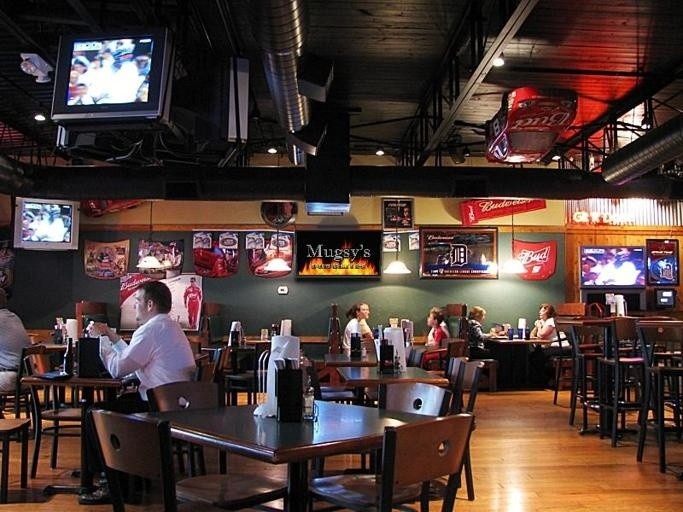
[80,486,113,505]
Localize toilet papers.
[227,322,242,346]
[281,320,291,335]
[65,319,79,344]
[253,335,300,417]
[517,317,527,338]
[384,327,407,372]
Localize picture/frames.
[380,197,415,233]
[418,224,499,281]
[644,238,680,288]
[292,223,383,280]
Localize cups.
[261,329,268,340]
[518,328,523,339]
[509,329,513,340]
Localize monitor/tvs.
[577,245,646,290]
[12,194,81,251]
[50,24,168,122]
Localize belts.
[0,369,17,372]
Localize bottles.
[64,338,74,376]
[271,323,279,340]
[361,348,367,358]
[378,325,384,340]
[525,329,530,340]
[373,327,379,339]
[241,337,246,345]
[303,386,315,420]
[328,304,340,355]
[394,356,401,369]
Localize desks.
[22,369,134,497]
[126,402,441,512]
[336,364,450,388]
[504,350,507,352]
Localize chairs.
[376,380,451,417]
[307,359,357,405]
[552,302,681,478]
[85,408,288,512]
[184,303,256,391]
[0,344,87,503]
[423,304,495,393]
[309,303,378,386]
[445,355,481,502]
[309,411,474,512]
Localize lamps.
[382,252,412,276]
[266,146,277,154]
[374,146,385,158]
[551,151,562,162]
[34,111,46,122]
[493,55,505,71]
[641,117,653,130]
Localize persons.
[184,277,201,330]
[0,287,35,392]
[422,306,451,368]
[340,302,374,356]
[401,207,411,227]
[272,202,293,225]
[21,204,66,242]
[465,305,496,360]
[528,303,570,390]
[67,38,152,104]
[78,281,195,505]
[582,248,643,286]
